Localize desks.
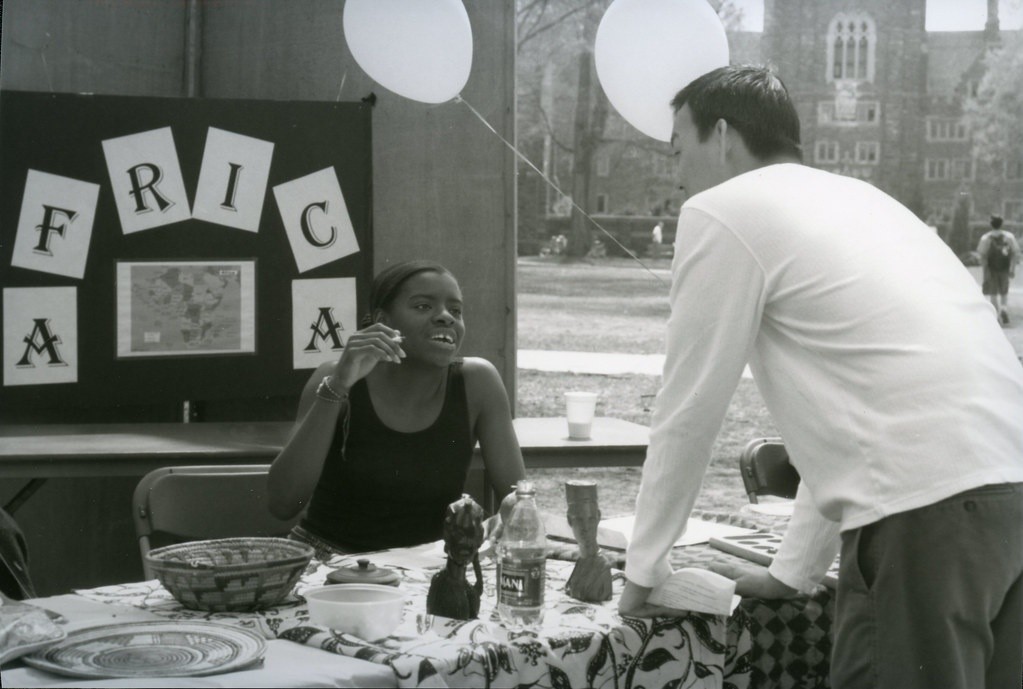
[1,415,651,495]
[0,493,844,689]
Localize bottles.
[496,481,545,634]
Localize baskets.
[146,535,316,611]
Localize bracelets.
[315,375,351,463]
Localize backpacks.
[989,235,1011,270]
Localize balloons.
[594,0,729,142]
[342,0,473,104]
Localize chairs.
[739,436,801,504]
[133,464,274,582]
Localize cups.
[564,392,597,438]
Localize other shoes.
[1000,306,1010,326]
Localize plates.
[21,619,268,678]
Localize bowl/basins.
[145,537,315,612]
[303,584,408,642]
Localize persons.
[426,496,484,620]
[651,221,664,244]
[619,64,1023,689]
[975,214,1019,325]
[266,259,527,560]
[565,482,613,604]
